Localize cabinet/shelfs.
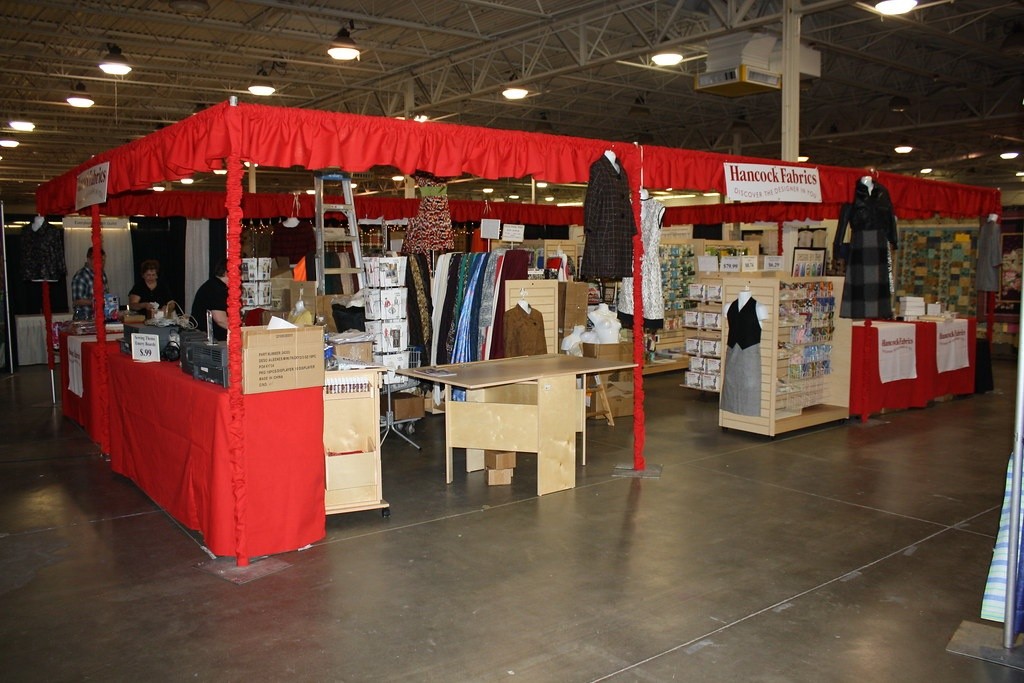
[646,236,853,437]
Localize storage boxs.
[330,340,375,366]
[381,393,425,418]
[230,326,326,396]
[582,342,634,419]
[485,468,514,486]
[484,451,518,469]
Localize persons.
[616,189,667,320]
[17,216,67,283]
[580,150,638,279]
[397,172,456,254]
[70,246,111,321]
[187,257,229,342]
[502,302,548,358]
[832,175,898,319]
[270,216,314,264]
[285,301,314,326]
[718,289,770,419]
[975,213,1004,293]
[128,259,178,319]
[559,304,623,357]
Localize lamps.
[502,73,528,99]
[248,67,277,96]
[651,35,684,68]
[326,28,360,62]
[250,216,281,227]
[98,46,132,75]
[66,82,94,108]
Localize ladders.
[314,167,372,315]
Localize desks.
[849,316,979,423]
[394,352,639,496]
[323,367,389,517]
[15,313,74,367]
[56,330,329,567]
